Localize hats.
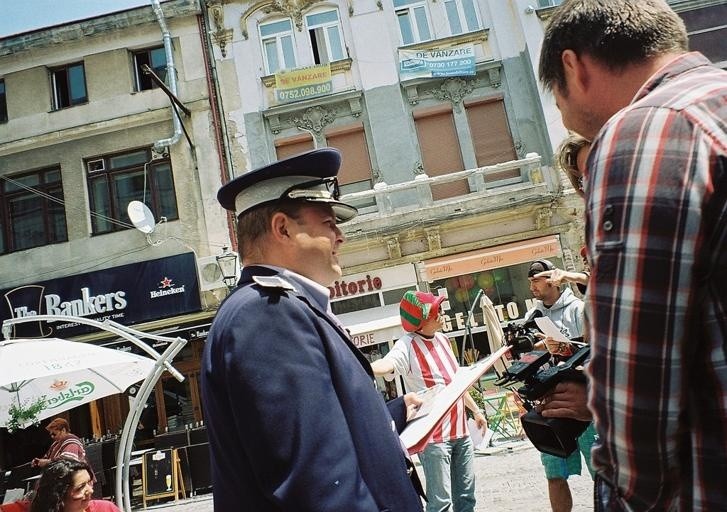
[215,143,360,227]
[398,289,448,334]
[526,258,558,278]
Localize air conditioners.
[196,251,242,292]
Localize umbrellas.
[0,313,187,512]
[461,287,515,446]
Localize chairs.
[0,424,210,494]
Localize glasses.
[49,431,61,436]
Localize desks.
[483,391,525,439]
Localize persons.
[369,289,488,512]
[536,0,727,512]
[198,145,426,512]
[533,246,592,287]
[30,417,98,487]
[557,134,592,198]
[0,459,122,512]
[524,258,599,512]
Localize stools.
[486,415,512,447]
